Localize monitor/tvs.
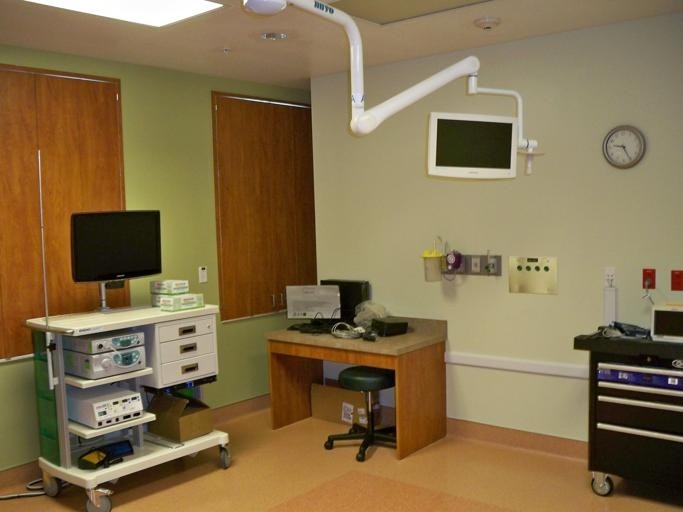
[71,210,161,283]
[286,285,341,333]
[650,304,683,343]
[427,111,517,179]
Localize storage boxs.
[309,379,378,429]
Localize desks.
[264,316,447,460]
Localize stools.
[323,366,396,463]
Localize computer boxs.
[321,280,370,328]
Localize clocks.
[601,123,646,170]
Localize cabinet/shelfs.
[574,331,682,508]
[22,303,232,511]
